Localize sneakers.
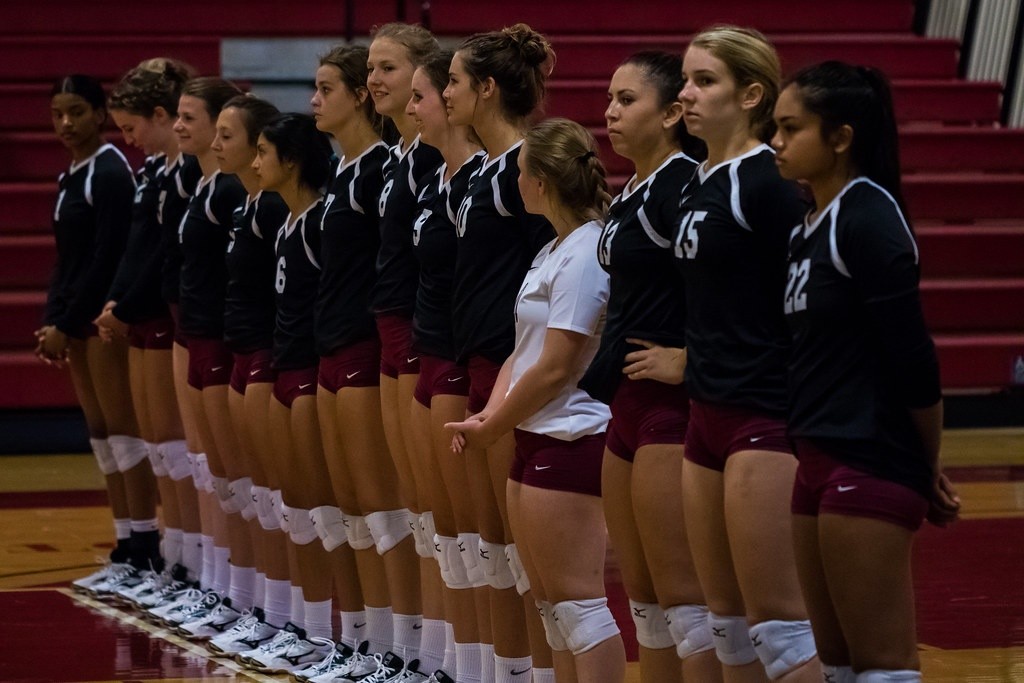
[74,562,454,683]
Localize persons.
[775,59,958,681]
[33,25,824,682]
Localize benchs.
[0,0,1023,407]
